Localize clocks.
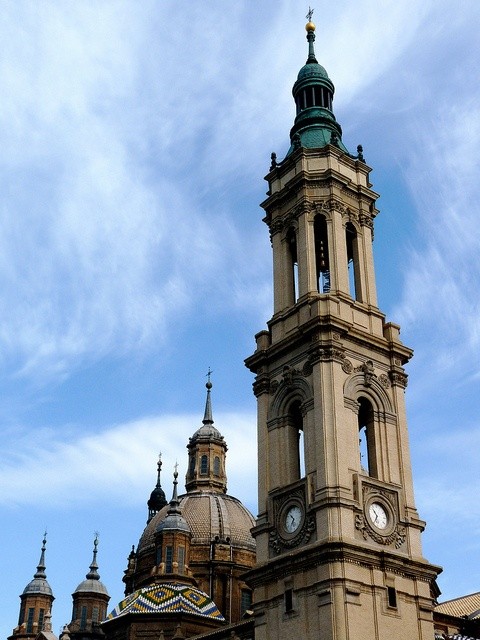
[368,503,390,531]
[283,506,302,534]
